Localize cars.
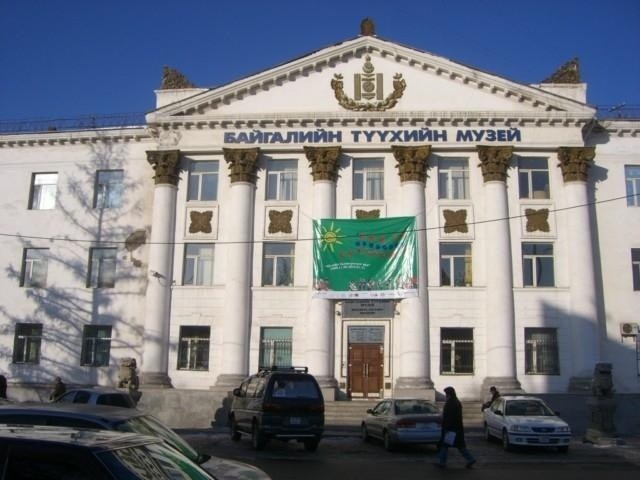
[483,396,572,453]
[0,387,270,480]
[362,399,445,452]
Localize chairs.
[413,405,422,411]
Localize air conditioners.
[620,322,638,337]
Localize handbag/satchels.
[444,431,456,445]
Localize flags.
[312,215,419,290]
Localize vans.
[228,365,325,451]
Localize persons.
[480,386,501,412]
[431,386,477,472]
